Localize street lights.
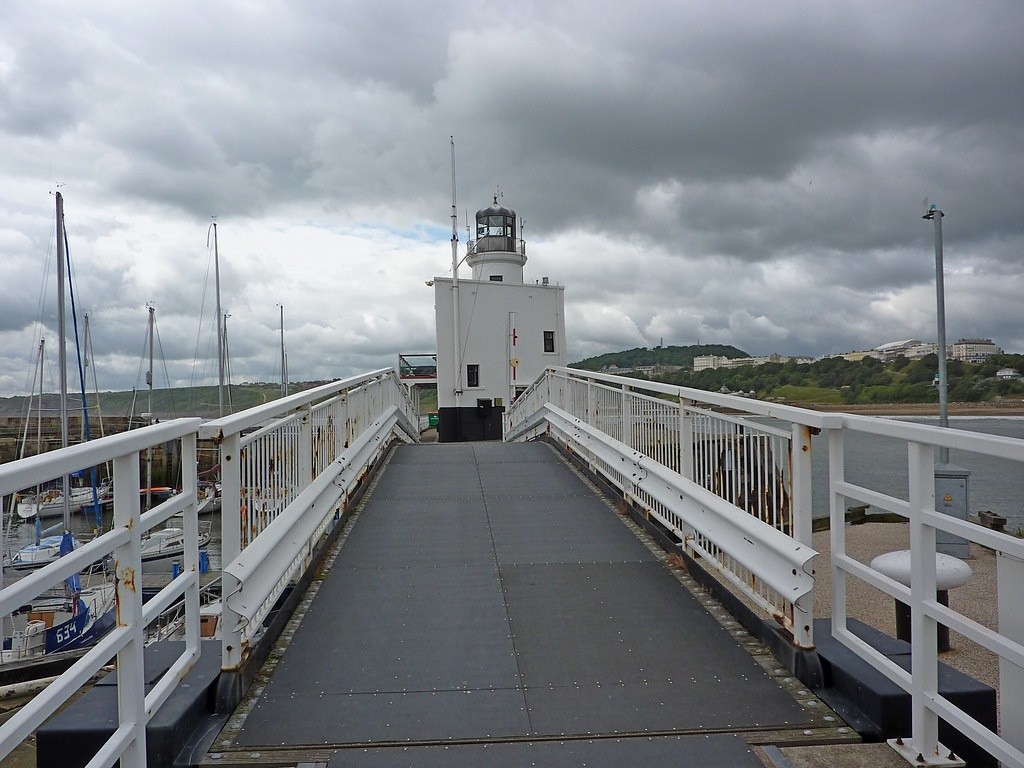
[921,203,952,464]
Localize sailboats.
[0,186,290,665]
